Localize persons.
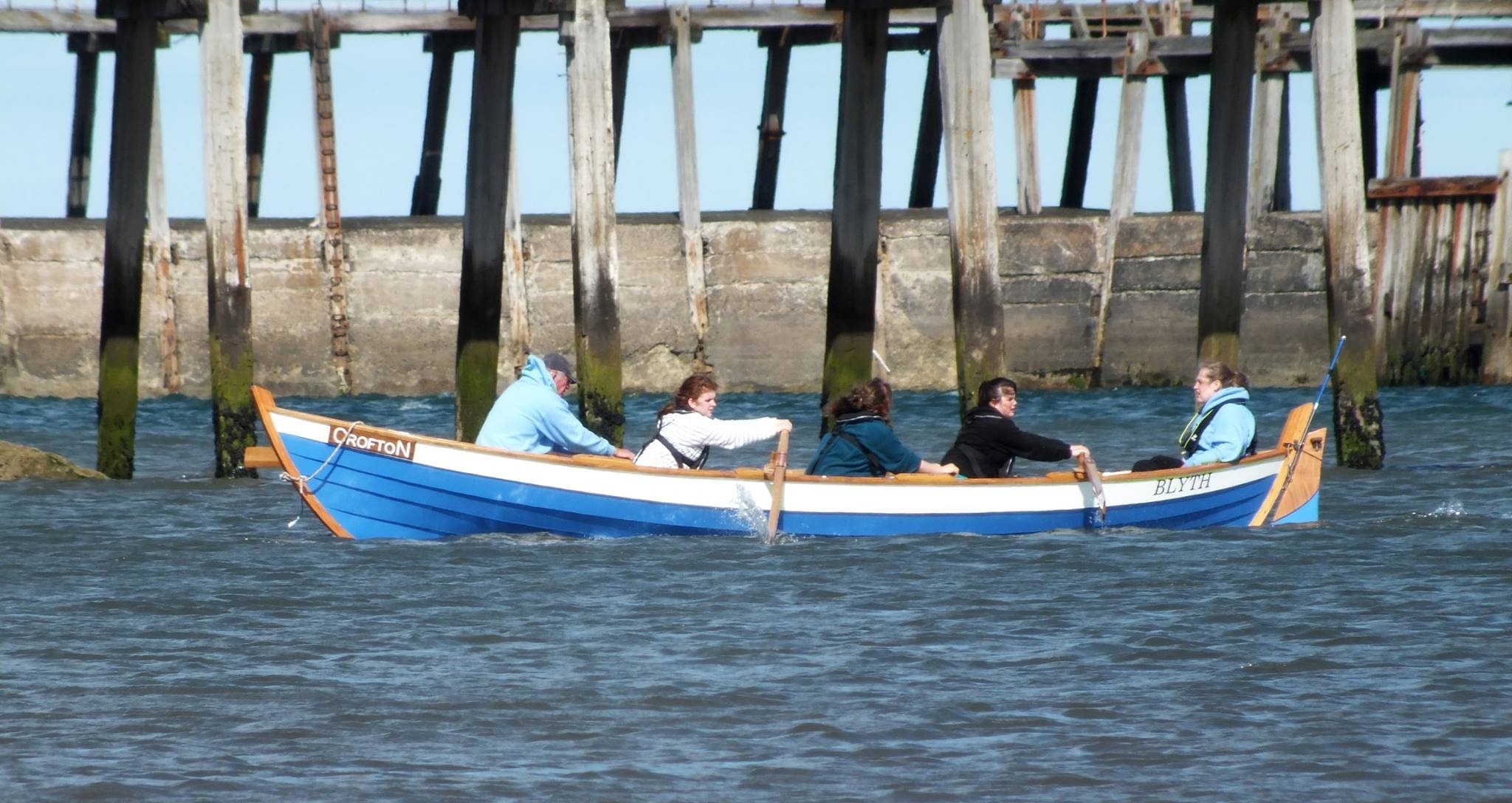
[803,378,958,478]
[941,378,1090,478]
[1129,362,1257,469]
[635,375,792,470]
[474,352,634,460]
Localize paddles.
[766,429,789,535]
[1261,335,1346,529]
[1081,451,1107,520]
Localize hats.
[542,352,582,386]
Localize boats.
[238,337,1348,543]
